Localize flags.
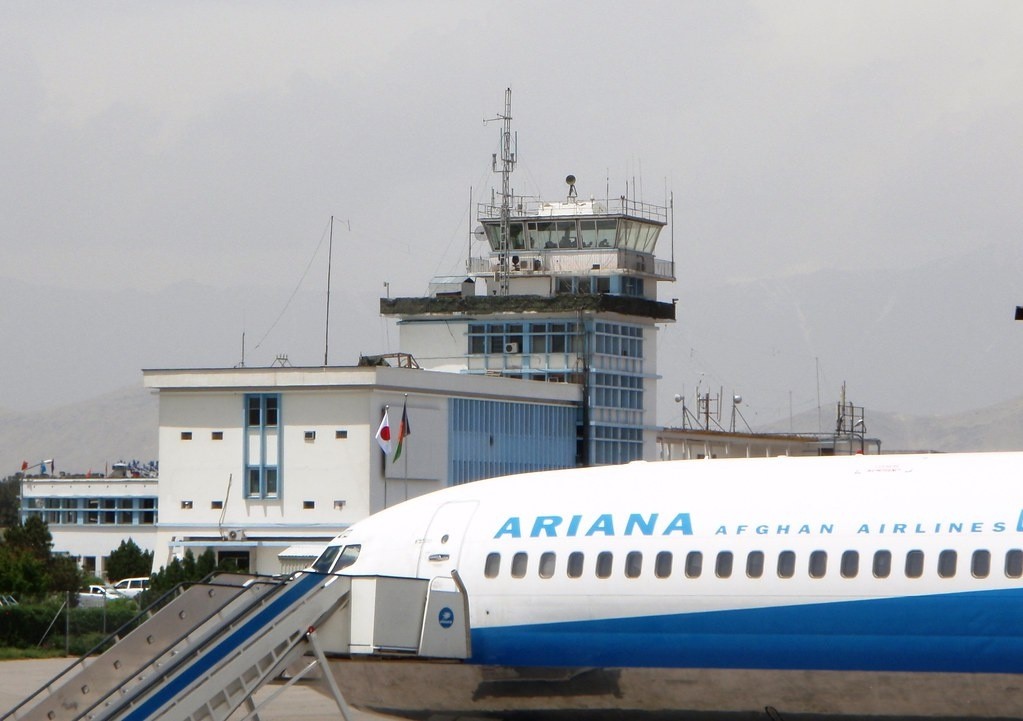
[86,469,91,477]
[40,463,46,474]
[22,462,27,470]
[392,403,411,463]
[51,460,54,472]
[375,410,391,455]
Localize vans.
[113,577,152,597]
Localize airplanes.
[288,452,1023,721]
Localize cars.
[76,585,125,606]
